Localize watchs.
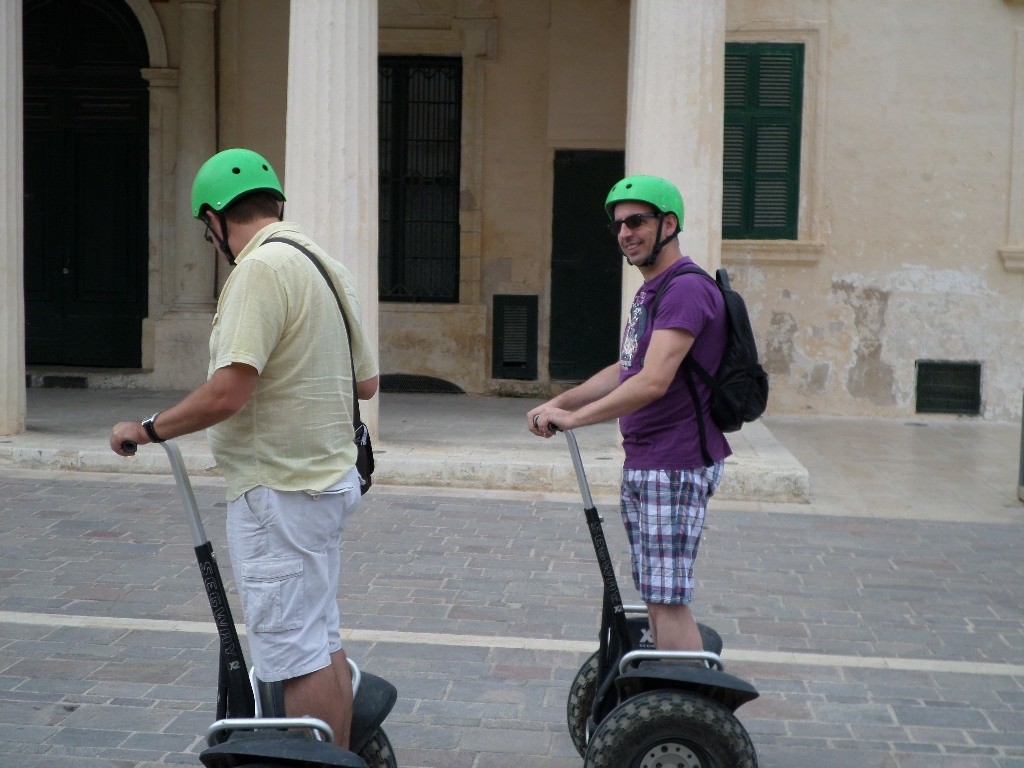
[142,413,167,443]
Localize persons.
[110,148,378,751]
[527,174,734,662]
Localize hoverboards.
[530,411,764,767]
[117,430,400,768]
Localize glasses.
[203,217,214,244]
[606,213,667,229]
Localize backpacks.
[650,269,768,434]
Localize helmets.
[604,174,684,231]
[190,147,287,219]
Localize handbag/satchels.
[353,417,375,494]
